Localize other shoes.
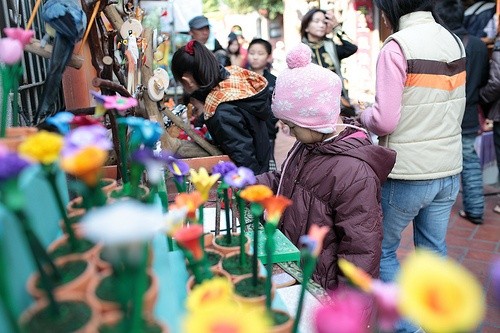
[493,205,500,213]
[460,209,482,224]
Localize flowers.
[0,27,487,333]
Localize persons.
[359,0,467,333]
[188,16,211,44]
[247,38,279,171]
[479,15,500,213]
[300,8,358,117]
[434,0,490,224]
[172,40,272,176]
[226,25,249,69]
[250,41,397,292]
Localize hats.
[271,43,369,135]
[212,38,226,52]
[188,15,209,29]
